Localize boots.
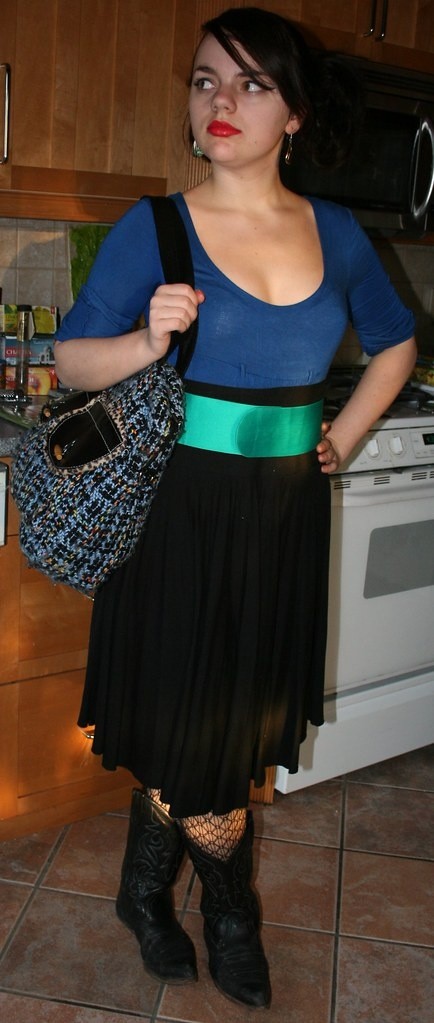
[170,815,272,1010]
[116,789,198,985]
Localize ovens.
[282,51,434,235]
[274,468,434,795]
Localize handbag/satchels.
[11,197,197,603]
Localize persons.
[53,9,416,1007]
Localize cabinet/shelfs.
[0,0,434,223]
[0,456,151,845]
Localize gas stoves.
[323,363,434,474]
